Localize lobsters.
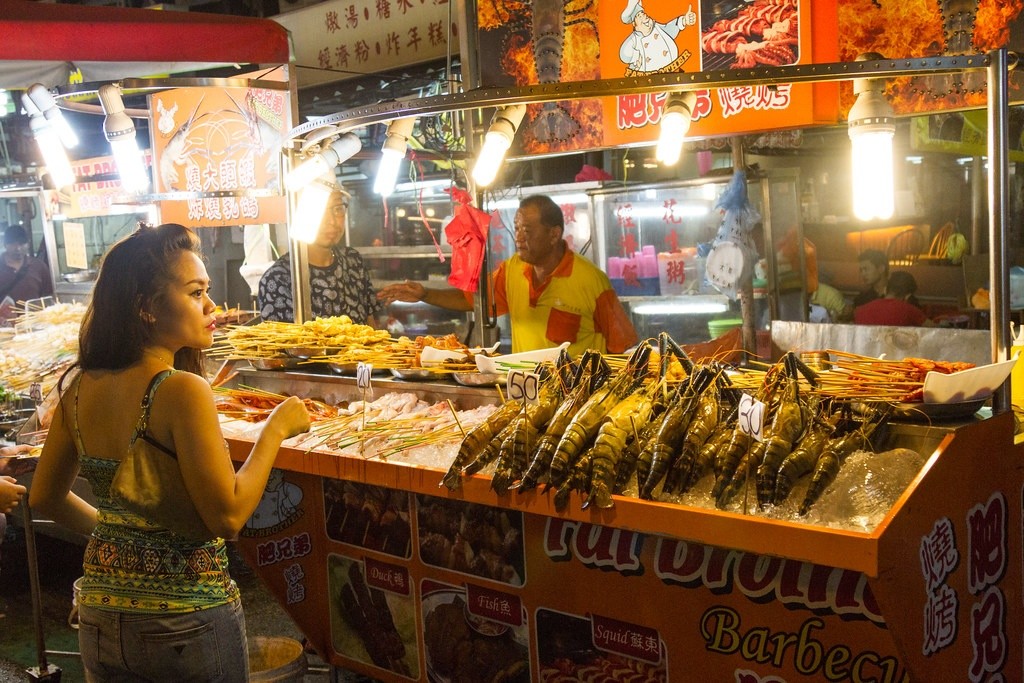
[158,89,284,194]
[437,328,894,509]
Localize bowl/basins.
[473,341,572,374]
[463,600,510,636]
[708,318,743,340]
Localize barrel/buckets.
[247,636,308,683]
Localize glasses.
[322,203,348,219]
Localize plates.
[868,392,992,419]
[61,271,96,283]
[421,590,528,683]
[248,346,510,387]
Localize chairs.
[907,221,956,264]
[886,228,924,267]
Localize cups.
[608,246,659,278]
[696,150,712,177]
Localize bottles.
[656,243,723,295]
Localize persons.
[0,444,34,514]
[854,270,935,327]
[0,225,53,317]
[376,195,638,358]
[258,179,383,330]
[27,223,311,683]
[853,249,920,307]
[809,281,846,325]
